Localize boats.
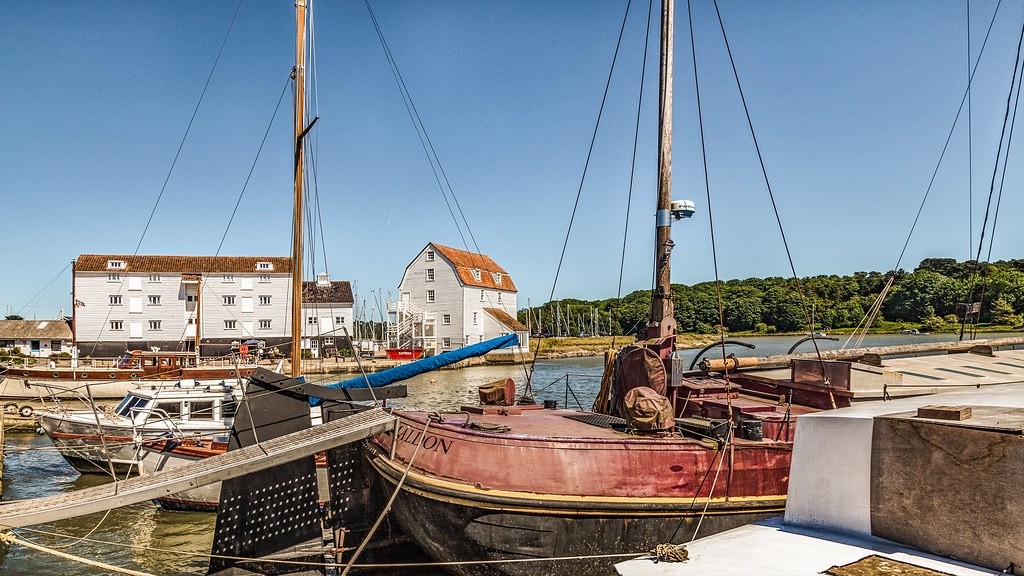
[30,379,245,474]
[131,434,227,513]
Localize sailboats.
[0,1,265,431]
[207,3,1023,575]
